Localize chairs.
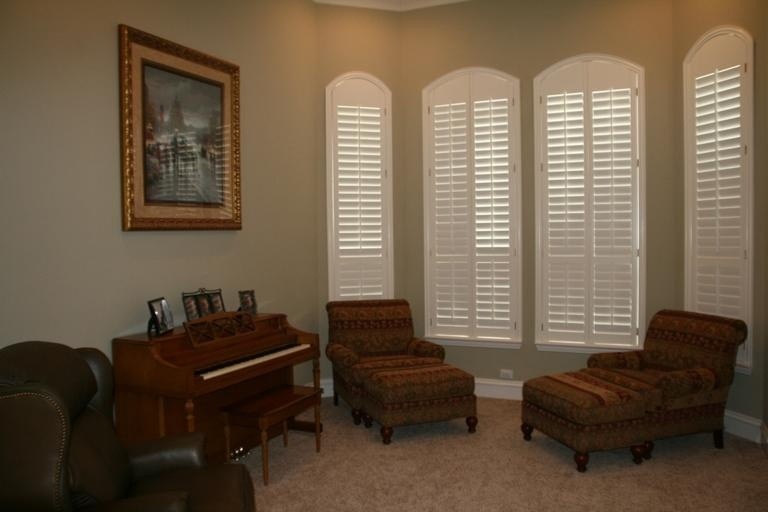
[0,341,254,512]
[579,308,748,460]
[325,299,445,424]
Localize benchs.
[219,385,324,485]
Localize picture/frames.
[147,297,174,335]
[238,290,257,315]
[119,23,241,231]
[182,288,225,322]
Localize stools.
[521,372,646,472]
[364,364,478,444]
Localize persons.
[198,296,213,317]
[185,297,197,320]
[241,293,255,315]
[210,293,225,314]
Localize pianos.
[111,312,322,465]
